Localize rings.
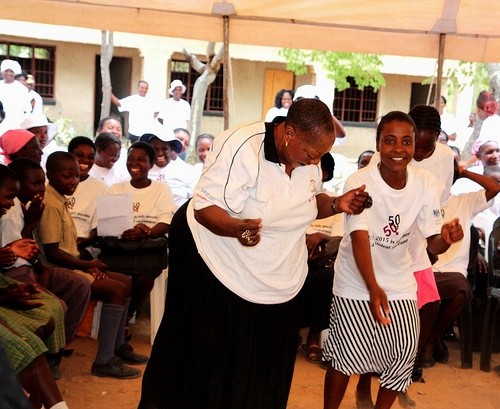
[359,193,373,210]
[242,229,253,243]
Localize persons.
[356,150,374,169]
[0,59,69,409]
[319,111,464,409]
[65,136,105,258]
[355,104,463,409]
[152,79,192,158]
[35,152,149,376]
[295,151,345,363]
[98,142,177,338]
[102,81,158,143]
[137,98,373,409]
[264,86,346,140]
[412,90,500,409]
[89,116,215,203]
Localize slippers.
[300,343,323,364]
[296,335,303,351]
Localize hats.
[26,74,35,84]
[139,126,183,154]
[0,59,23,76]
[15,70,28,80]
[20,113,55,130]
[292,85,320,101]
[168,79,186,96]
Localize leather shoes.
[90,355,142,379]
[116,342,150,364]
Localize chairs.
[479,228,500,372]
[458,226,479,368]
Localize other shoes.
[125,318,132,341]
[421,351,435,368]
[429,335,449,363]
[411,357,422,382]
[444,325,458,341]
[48,361,61,380]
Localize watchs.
[318,241,326,256]
[331,196,340,215]
